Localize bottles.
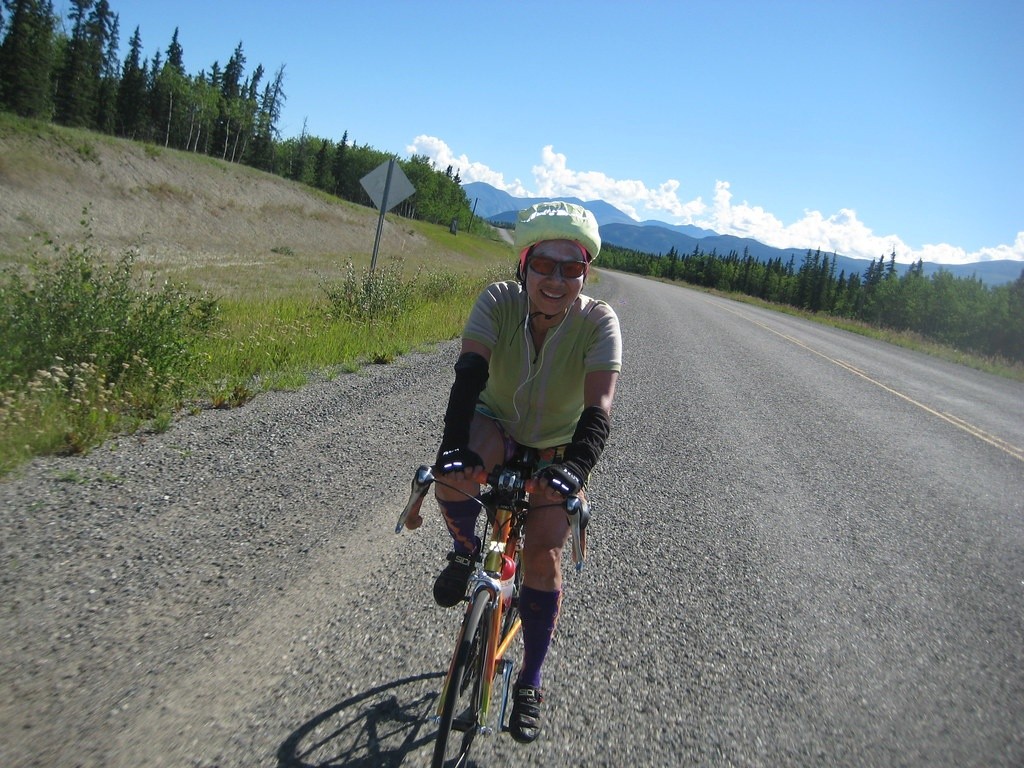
[498,554,517,614]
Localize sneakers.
[509,669,545,743]
[432,534,481,608]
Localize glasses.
[526,254,587,279]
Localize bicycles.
[394,447,592,767]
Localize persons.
[434,200,623,744]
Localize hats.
[514,200,602,261]
